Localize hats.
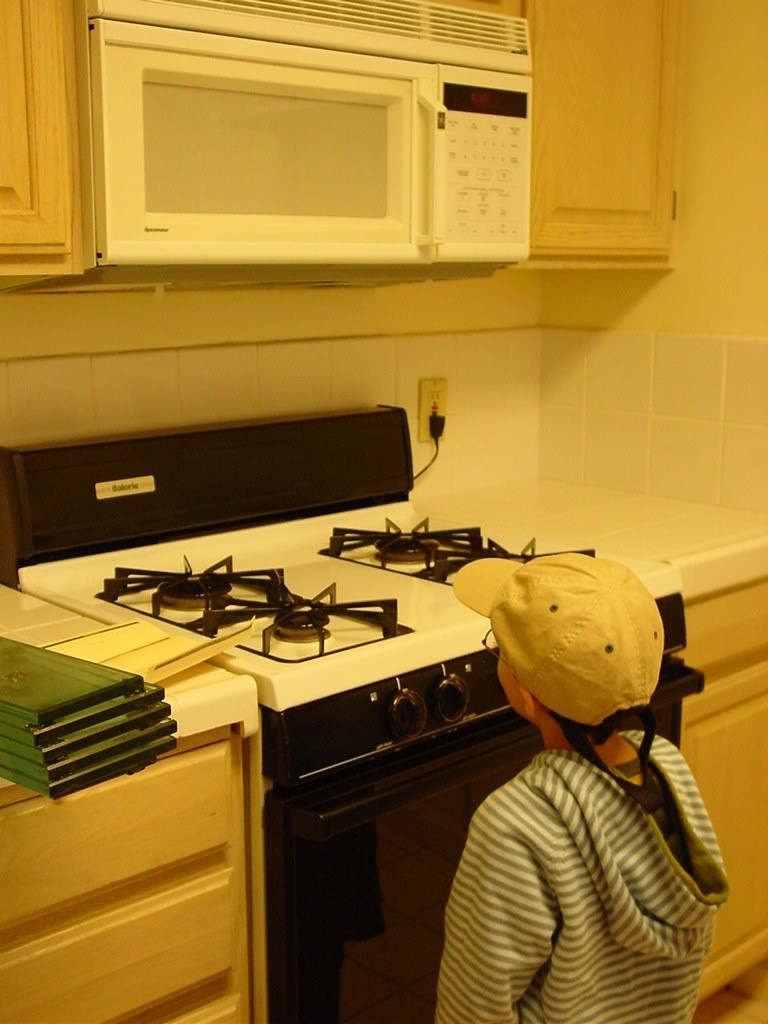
[452,557,667,726]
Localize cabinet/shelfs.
[0,723,255,1024]
[0,1,83,290]
[681,575,768,1006]
[518,0,684,272]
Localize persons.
[435,549,735,1023]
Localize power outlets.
[419,379,447,442]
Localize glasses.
[482,627,508,666]
